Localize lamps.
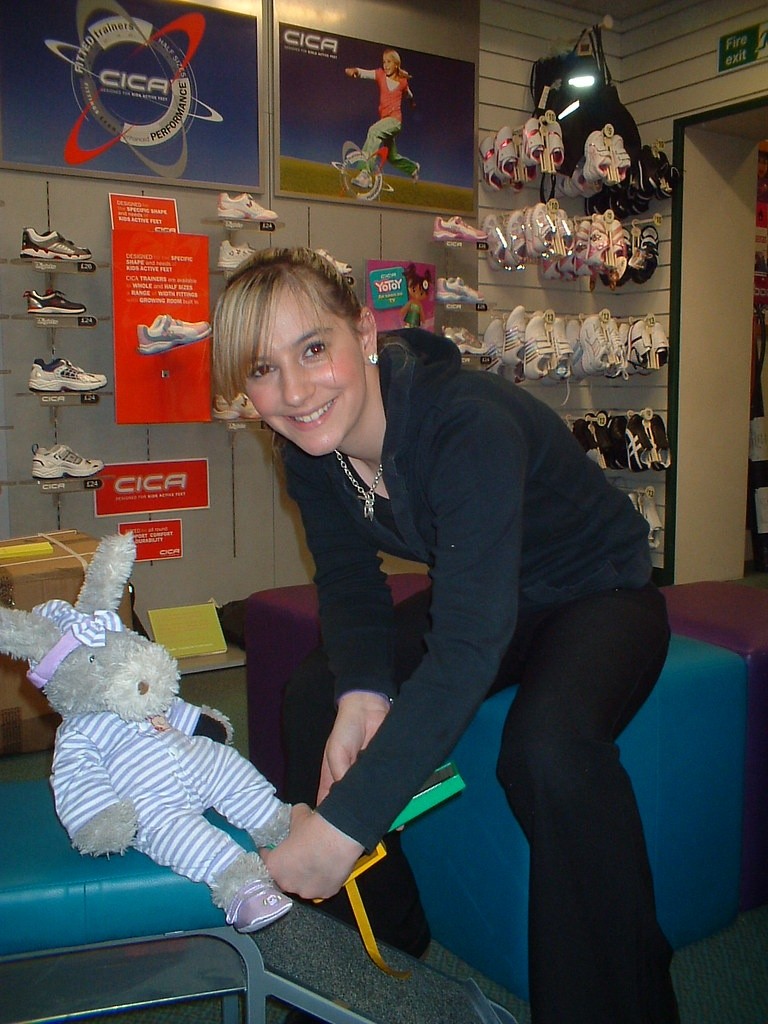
[568,54,597,88]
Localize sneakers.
[137,314,211,354]
[212,392,262,420]
[484,202,660,287]
[23,289,86,314]
[436,277,485,302]
[411,163,420,184]
[480,118,680,220]
[433,216,487,241]
[28,357,108,392]
[32,444,104,480]
[351,170,373,189]
[217,240,257,268]
[20,226,91,261]
[443,325,488,355]
[627,486,663,549]
[218,192,278,221]
[484,307,670,407]
[568,410,671,473]
[316,249,352,274]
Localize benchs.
[0,776,520,1024]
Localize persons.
[211,246,681,1024]
[346,48,420,189]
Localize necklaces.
[334,449,383,523]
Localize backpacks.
[529,25,641,190]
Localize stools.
[239,576,767,1004]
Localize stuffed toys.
[0,535,292,932]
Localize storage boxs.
[0,530,134,755]
